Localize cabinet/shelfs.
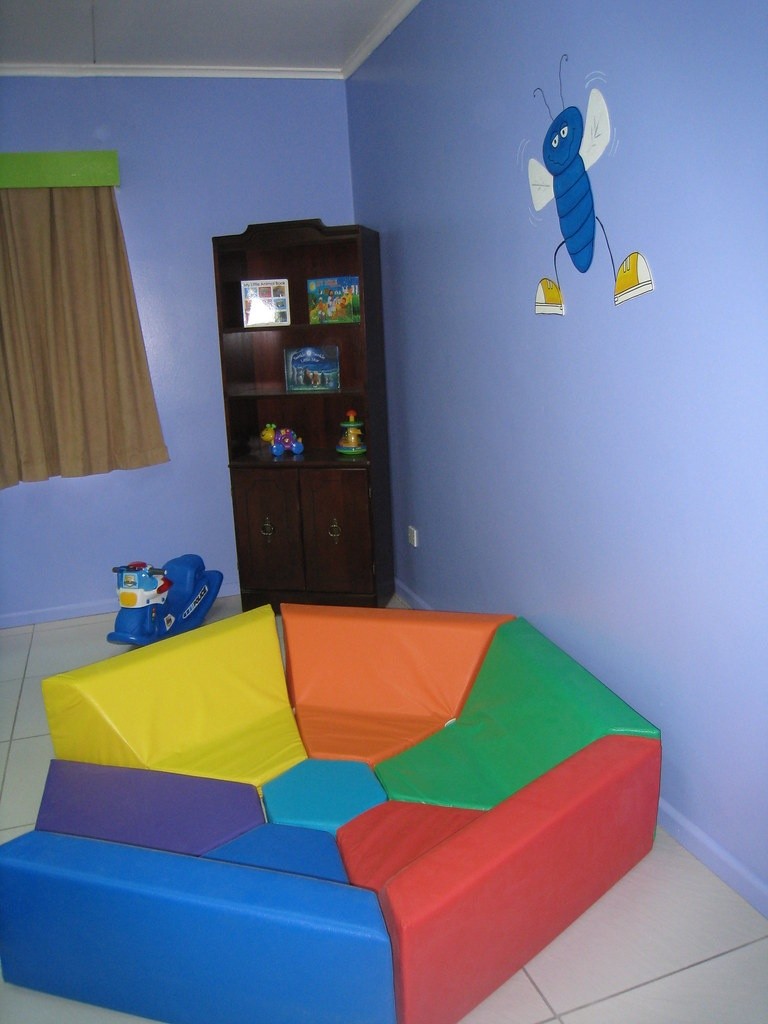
[211,219,395,613]
